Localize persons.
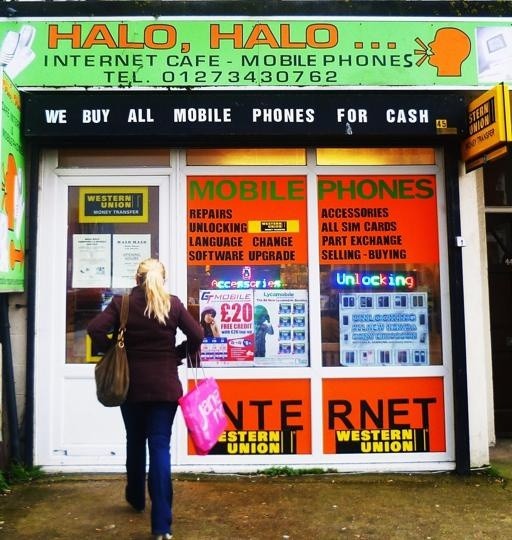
[200,306,219,337]
[87,257,204,539]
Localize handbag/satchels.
[92,293,130,408]
[178,376,228,451]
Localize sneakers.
[153,532,173,540]
[125,485,145,513]
